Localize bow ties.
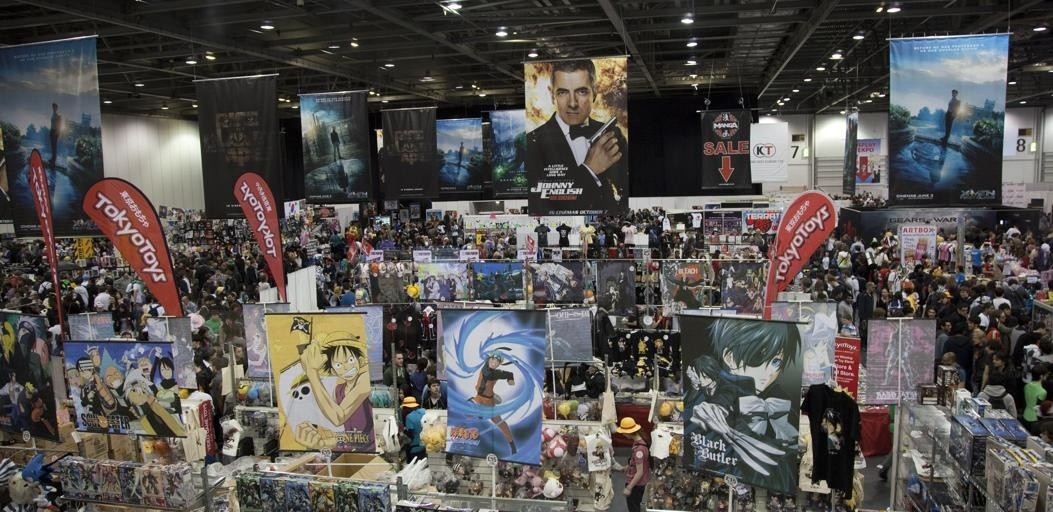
[569,125,594,140]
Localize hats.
[334,287,340,294]
[617,417,641,434]
[187,313,205,329]
[407,284,421,298]
[866,281,877,288]
[659,403,671,417]
[64,256,72,263]
[216,287,225,295]
[371,262,404,278]
[400,396,419,408]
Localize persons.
[525,59,628,214]
[45,103,63,174]
[328,126,343,163]
[939,90,961,150]
[455,140,466,172]
[1,190,1053,511]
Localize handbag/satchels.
[648,454,654,470]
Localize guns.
[589,116,616,147]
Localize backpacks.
[544,363,605,399]
[985,389,1008,409]
[40,282,50,301]
[63,291,85,314]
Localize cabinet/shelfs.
[194,203,1051,511]
